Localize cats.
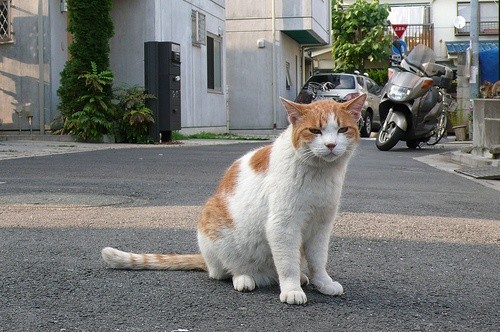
[101,93,367,305]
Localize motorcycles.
[375,40,454,152]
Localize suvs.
[293,70,381,138]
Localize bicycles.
[426,88,452,146]
